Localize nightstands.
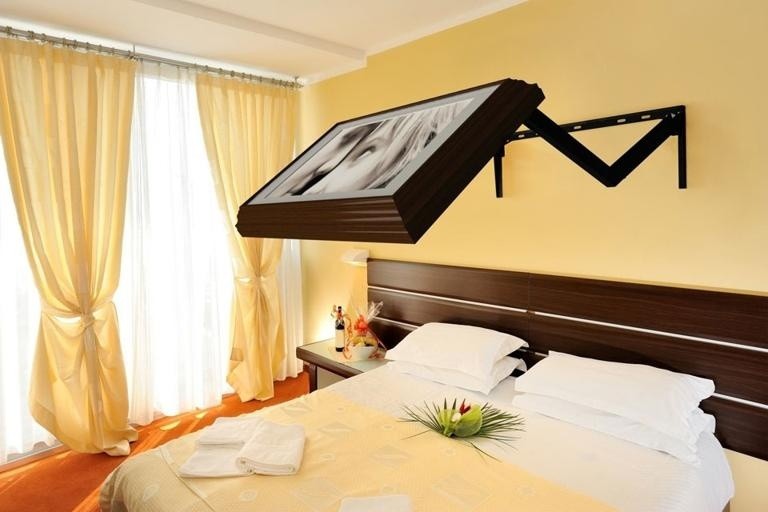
[294,335,389,392]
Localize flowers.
[392,396,527,465]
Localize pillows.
[389,355,527,396]
[384,320,530,380]
[513,349,716,442]
[510,394,716,465]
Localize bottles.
[334,306,345,353]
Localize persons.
[280,122,372,195]
[302,99,474,196]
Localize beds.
[96,255,768,511]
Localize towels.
[235,422,306,476]
[176,446,247,478]
[200,416,262,448]
[338,493,411,511]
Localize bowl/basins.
[346,346,377,362]
